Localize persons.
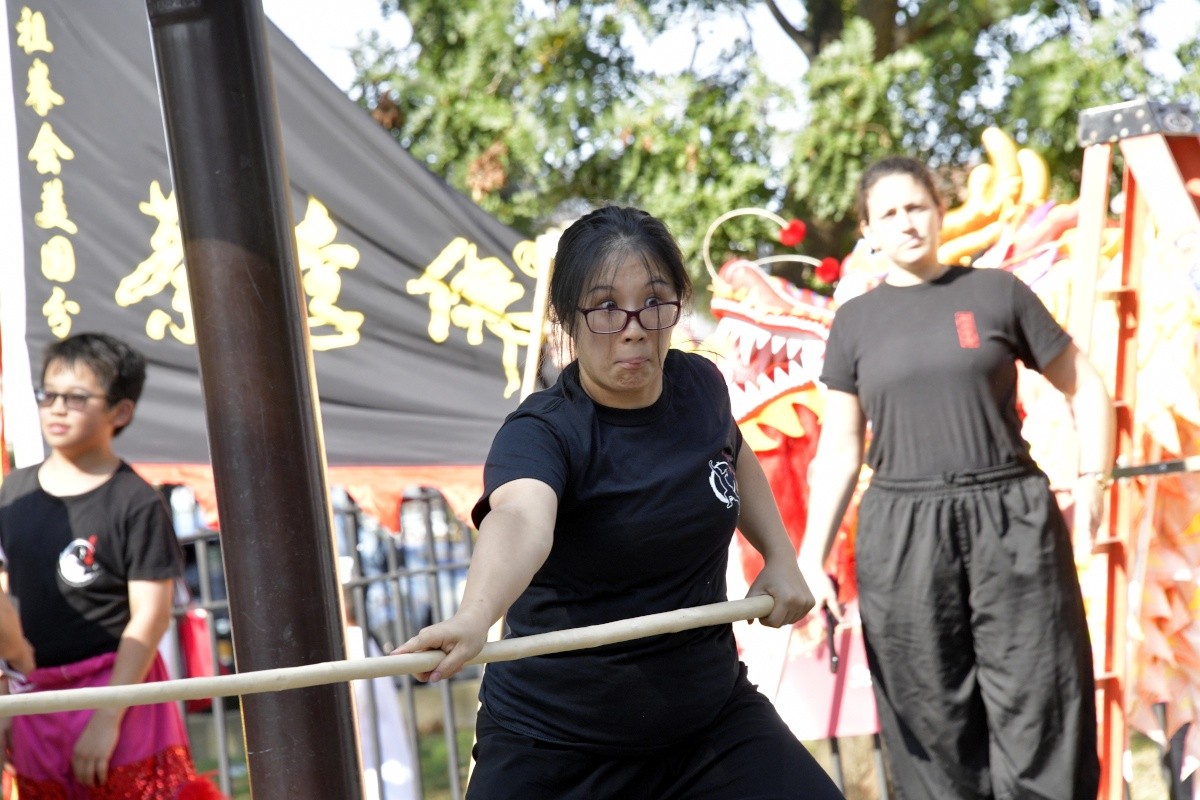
[797,157,1115,800]
[389,212,846,800]
[1,333,220,800]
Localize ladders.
[1066,98,1200,800]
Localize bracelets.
[1078,472,1111,492]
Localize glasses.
[33,387,109,410]
[576,298,681,333]
